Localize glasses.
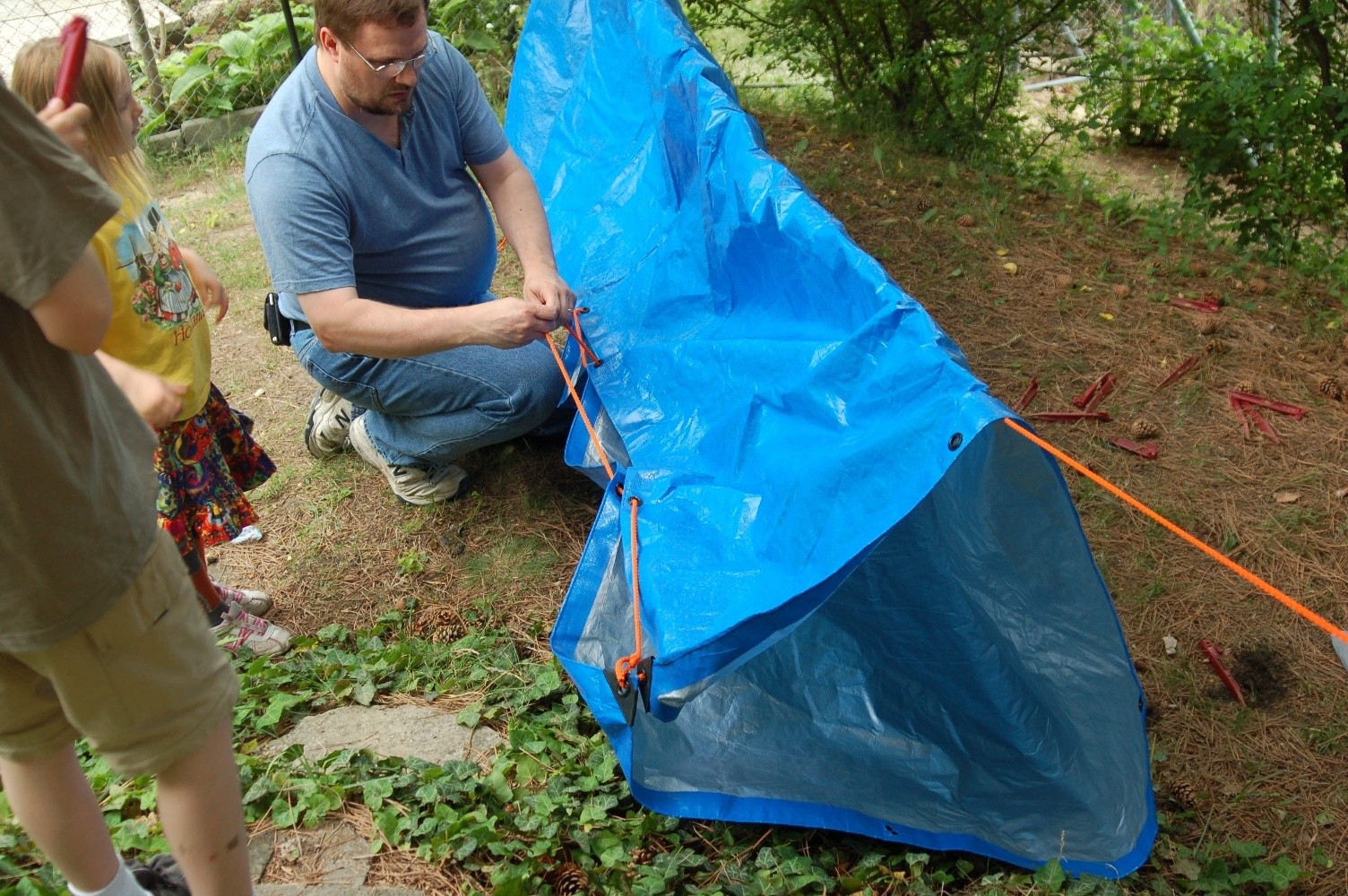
[344,28,438,80]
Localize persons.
[0,72,256,896]
[243,0,579,506]
[10,14,296,663]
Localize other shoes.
[129,853,192,896]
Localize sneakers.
[304,385,352,461]
[217,586,273,616]
[208,601,294,661]
[348,413,470,505]
